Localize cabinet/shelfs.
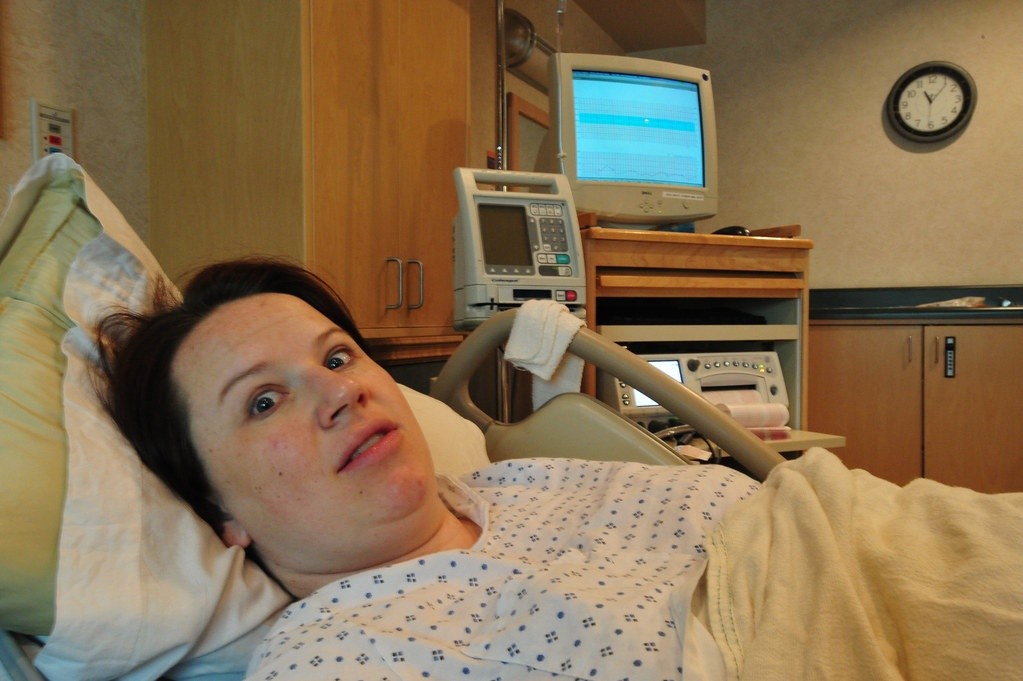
[142,0,472,338]
[508,211,849,461]
[807,306,1023,494]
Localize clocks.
[884,60,977,145]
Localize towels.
[503,297,587,413]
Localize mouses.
[712,225,754,237]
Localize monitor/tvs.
[549,52,719,231]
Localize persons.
[97,258,764,681]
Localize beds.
[0,309,787,681]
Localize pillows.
[0,154,491,679]
